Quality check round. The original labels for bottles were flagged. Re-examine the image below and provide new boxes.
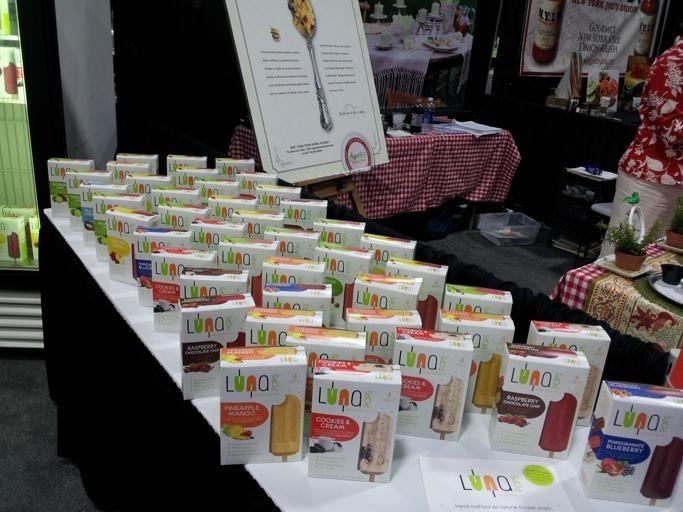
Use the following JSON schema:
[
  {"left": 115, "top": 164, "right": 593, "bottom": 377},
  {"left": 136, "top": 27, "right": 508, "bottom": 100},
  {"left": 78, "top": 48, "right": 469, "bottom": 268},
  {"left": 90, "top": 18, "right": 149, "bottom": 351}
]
[
  {"left": 413, "top": 98, "right": 433, "bottom": 133},
  {"left": 634, "top": 0, "right": 661, "bottom": 56},
  {"left": 531, "top": 1, "right": 566, "bottom": 64}
]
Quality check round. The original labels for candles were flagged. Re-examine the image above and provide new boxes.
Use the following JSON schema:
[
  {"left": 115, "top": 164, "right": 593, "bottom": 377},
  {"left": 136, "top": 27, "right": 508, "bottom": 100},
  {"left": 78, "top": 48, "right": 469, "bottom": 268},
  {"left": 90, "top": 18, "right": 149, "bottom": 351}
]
[
  {"left": 375, "top": 4, "right": 384, "bottom": 16},
  {"left": 431, "top": 2, "right": 440, "bottom": 16},
  {"left": 396, "top": 0, "right": 404, "bottom": 5}
]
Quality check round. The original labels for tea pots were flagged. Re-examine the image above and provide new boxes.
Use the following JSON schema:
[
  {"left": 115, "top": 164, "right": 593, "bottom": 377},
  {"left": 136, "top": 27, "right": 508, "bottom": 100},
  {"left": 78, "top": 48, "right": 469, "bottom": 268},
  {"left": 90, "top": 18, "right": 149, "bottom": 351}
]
[{"left": 456, "top": 5, "right": 475, "bottom": 38}]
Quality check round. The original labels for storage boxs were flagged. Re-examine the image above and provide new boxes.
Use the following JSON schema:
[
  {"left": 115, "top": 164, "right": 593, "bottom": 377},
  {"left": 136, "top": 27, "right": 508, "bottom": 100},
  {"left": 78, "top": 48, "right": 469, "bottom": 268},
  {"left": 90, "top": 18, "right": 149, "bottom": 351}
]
[{"left": 478, "top": 213, "right": 542, "bottom": 248}]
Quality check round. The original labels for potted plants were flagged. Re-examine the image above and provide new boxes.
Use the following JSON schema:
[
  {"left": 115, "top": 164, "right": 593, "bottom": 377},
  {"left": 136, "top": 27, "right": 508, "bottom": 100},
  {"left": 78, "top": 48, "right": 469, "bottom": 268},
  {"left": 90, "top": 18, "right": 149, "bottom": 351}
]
[
  {"left": 661, "top": 194, "right": 683, "bottom": 249},
  {"left": 598, "top": 217, "right": 663, "bottom": 271}
]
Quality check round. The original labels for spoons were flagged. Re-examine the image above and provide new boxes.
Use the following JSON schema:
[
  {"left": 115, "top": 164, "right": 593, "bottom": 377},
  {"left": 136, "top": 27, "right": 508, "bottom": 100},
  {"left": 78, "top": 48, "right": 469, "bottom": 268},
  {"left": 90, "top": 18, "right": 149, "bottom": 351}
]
[{"left": 288, "top": 0, "right": 333, "bottom": 131}]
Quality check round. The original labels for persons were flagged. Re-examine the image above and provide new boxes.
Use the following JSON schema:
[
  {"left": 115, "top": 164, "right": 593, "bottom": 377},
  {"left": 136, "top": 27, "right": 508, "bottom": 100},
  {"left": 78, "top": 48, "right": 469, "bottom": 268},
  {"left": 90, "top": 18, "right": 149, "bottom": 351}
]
[{"left": 596, "top": 31, "right": 683, "bottom": 260}]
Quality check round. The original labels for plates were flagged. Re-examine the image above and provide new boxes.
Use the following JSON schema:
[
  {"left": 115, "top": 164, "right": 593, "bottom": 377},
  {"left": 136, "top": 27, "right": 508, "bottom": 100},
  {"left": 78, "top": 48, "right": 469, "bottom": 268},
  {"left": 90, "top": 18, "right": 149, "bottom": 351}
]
[
  {"left": 362, "top": 22, "right": 385, "bottom": 35},
  {"left": 422, "top": 35, "right": 458, "bottom": 52}
]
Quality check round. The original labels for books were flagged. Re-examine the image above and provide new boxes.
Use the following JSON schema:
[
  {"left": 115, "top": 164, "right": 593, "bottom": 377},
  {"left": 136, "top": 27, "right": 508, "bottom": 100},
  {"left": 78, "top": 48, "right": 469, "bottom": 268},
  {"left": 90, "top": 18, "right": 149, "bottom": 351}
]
[{"left": 450, "top": 118, "right": 502, "bottom": 138}]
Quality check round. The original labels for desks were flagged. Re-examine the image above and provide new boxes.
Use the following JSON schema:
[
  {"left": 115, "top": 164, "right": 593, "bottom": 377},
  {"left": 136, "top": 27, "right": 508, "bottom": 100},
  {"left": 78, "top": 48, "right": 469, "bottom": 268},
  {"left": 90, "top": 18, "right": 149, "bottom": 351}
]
[
  {"left": 236, "top": 121, "right": 509, "bottom": 231},
  {"left": 555, "top": 236, "right": 683, "bottom": 356},
  {"left": 42, "top": 201, "right": 679, "bottom": 511}
]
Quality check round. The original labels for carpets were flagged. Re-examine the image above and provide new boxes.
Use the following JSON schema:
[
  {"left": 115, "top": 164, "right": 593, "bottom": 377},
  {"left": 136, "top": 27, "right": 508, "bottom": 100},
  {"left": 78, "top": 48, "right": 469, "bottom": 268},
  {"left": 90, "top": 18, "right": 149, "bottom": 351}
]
[{"left": 418, "top": 229, "right": 577, "bottom": 295}]
